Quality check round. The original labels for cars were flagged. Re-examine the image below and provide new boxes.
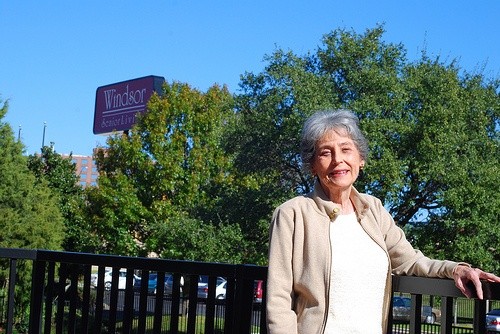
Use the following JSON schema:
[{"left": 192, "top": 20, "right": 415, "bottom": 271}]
[
  {"left": 90, "top": 268, "right": 142, "bottom": 295},
  {"left": 390, "top": 296, "right": 442, "bottom": 326},
  {"left": 486, "top": 307, "right": 500, "bottom": 334},
  {"left": 164, "top": 275, "right": 184, "bottom": 298},
  {"left": 133, "top": 273, "right": 168, "bottom": 298},
  {"left": 197, "top": 276, "right": 227, "bottom": 301}
]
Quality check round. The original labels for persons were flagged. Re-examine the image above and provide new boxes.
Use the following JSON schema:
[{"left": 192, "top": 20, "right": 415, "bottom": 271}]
[{"left": 264, "top": 111, "right": 500, "bottom": 334}]
